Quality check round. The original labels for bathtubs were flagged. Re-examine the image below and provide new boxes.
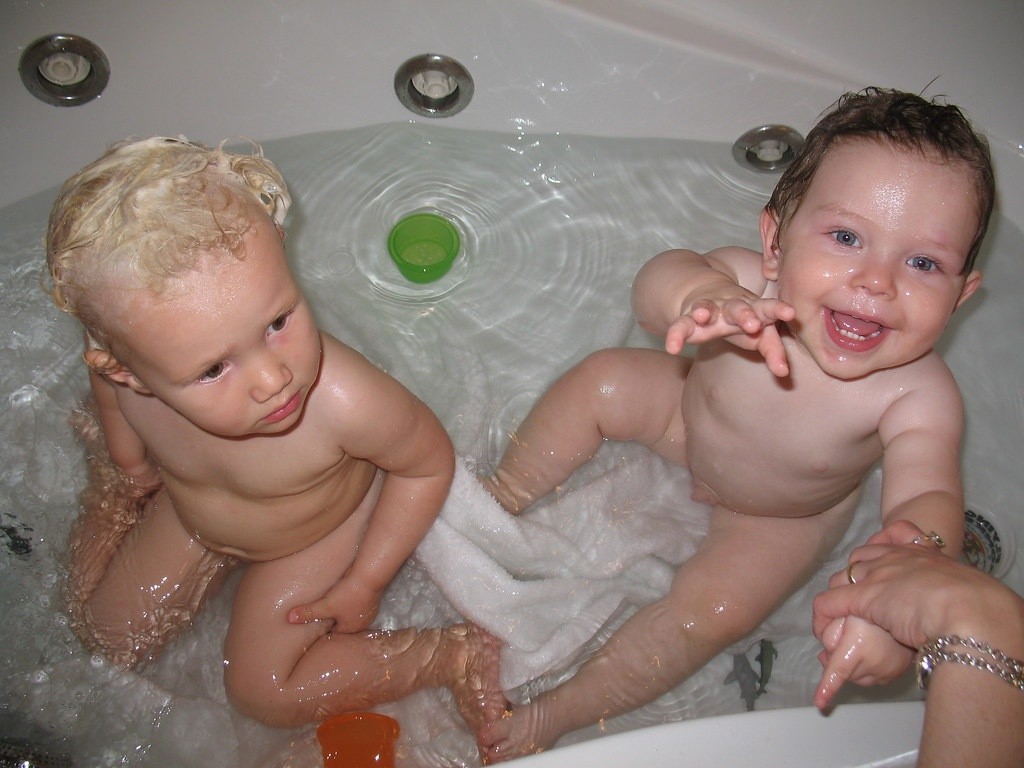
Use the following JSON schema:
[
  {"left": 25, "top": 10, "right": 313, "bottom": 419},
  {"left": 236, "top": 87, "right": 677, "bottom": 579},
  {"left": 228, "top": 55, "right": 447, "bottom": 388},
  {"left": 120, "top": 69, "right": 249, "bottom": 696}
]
[{"left": 2, "top": 0, "right": 1024, "bottom": 768}]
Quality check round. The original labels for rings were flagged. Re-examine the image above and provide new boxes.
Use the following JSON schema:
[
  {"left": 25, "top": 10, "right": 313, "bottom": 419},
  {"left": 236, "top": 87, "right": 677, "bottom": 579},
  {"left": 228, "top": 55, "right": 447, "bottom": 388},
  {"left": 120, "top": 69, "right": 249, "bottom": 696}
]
[
  {"left": 848, "top": 560, "right": 859, "bottom": 583},
  {"left": 911, "top": 532, "right": 944, "bottom": 547}
]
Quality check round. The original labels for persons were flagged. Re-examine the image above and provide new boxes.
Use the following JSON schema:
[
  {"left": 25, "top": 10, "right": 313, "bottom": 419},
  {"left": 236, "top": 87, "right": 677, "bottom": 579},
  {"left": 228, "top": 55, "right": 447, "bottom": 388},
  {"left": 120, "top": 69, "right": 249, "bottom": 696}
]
[
  {"left": 814, "top": 519, "right": 1024, "bottom": 768},
  {"left": 46, "top": 137, "right": 508, "bottom": 734},
  {"left": 472, "top": 84, "right": 996, "bottom": 767}
]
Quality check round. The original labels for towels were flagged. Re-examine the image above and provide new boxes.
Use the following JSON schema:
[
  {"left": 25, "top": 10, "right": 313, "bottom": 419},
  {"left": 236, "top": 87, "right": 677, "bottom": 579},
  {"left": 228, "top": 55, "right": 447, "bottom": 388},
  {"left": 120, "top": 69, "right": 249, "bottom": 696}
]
[{"left": 414, "top": 453, "right": 883, "bottom": 694}]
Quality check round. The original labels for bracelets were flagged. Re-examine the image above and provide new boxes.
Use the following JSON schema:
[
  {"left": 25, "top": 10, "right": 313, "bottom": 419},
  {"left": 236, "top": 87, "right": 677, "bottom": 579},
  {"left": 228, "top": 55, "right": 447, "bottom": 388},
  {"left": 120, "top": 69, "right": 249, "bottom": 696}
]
[{"left": 917, "top": 635, "right": 1023, "bottom": 697}]
